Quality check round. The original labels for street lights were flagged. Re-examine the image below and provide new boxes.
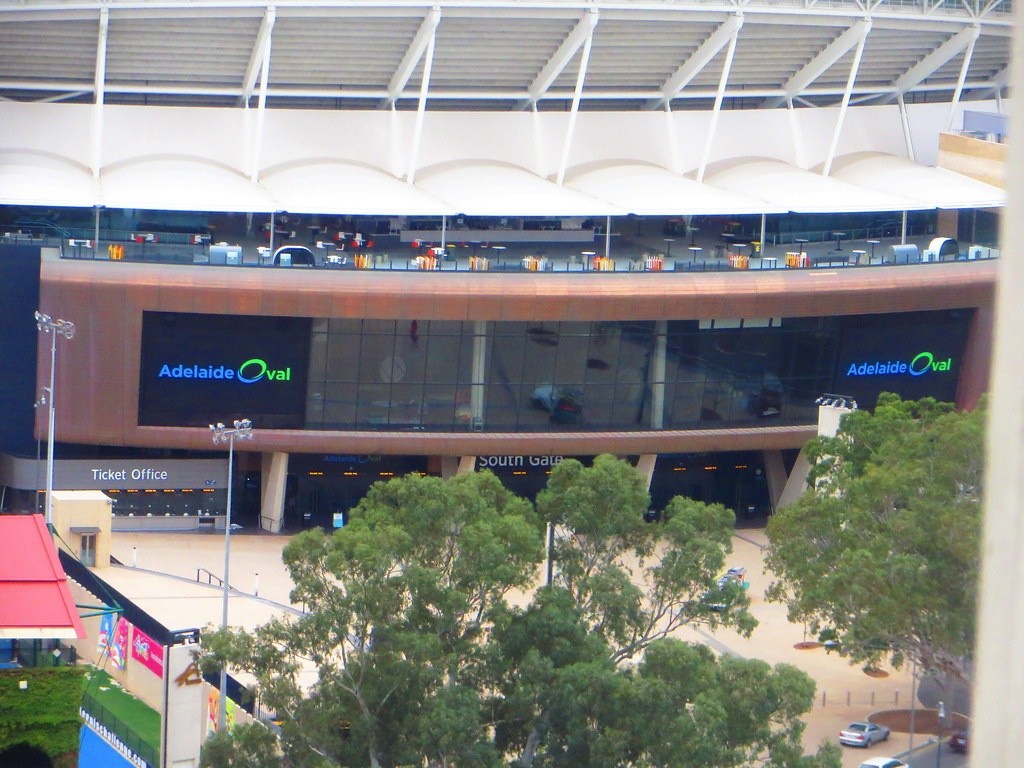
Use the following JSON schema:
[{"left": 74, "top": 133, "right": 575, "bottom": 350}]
[
  {"left": 821, "top": 640, "right": 917, "bottom": 768},
  {"left": 211, "top": 418, "right": 254, "bottom": 734},
  {"left": 33, "top": 310, "right": 76, "bottom": 524}
]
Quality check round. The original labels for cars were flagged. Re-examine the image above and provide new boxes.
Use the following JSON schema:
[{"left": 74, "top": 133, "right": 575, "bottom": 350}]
[
  {"left": 528, "top": 384, "right": 585, "bottom": 428},
  {"left": 948, "top": 730, "right": 968, "bottom": 754},
  {"left": 858, "top": 756, "right": 909, "bottom": 768},
  {"left": 702, "top": 566, "right": 746, "bottom": 612},
  {"left": 838, "top": 721, "right": 890, "bottom": 750}
]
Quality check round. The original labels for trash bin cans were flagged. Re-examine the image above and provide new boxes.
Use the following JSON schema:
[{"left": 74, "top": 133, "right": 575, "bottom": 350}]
[
  {"left": 208, "top": 243, "right": 243, "bottom": 265},
  {"left": 750, "top": 241, "right": 761, "bottom": 258},
  {"left": 280, "top": 253, "right": 291, "bottom": 267},
  {"left": 447, "top": 244, "right": 455, "bottom": 259}
]
